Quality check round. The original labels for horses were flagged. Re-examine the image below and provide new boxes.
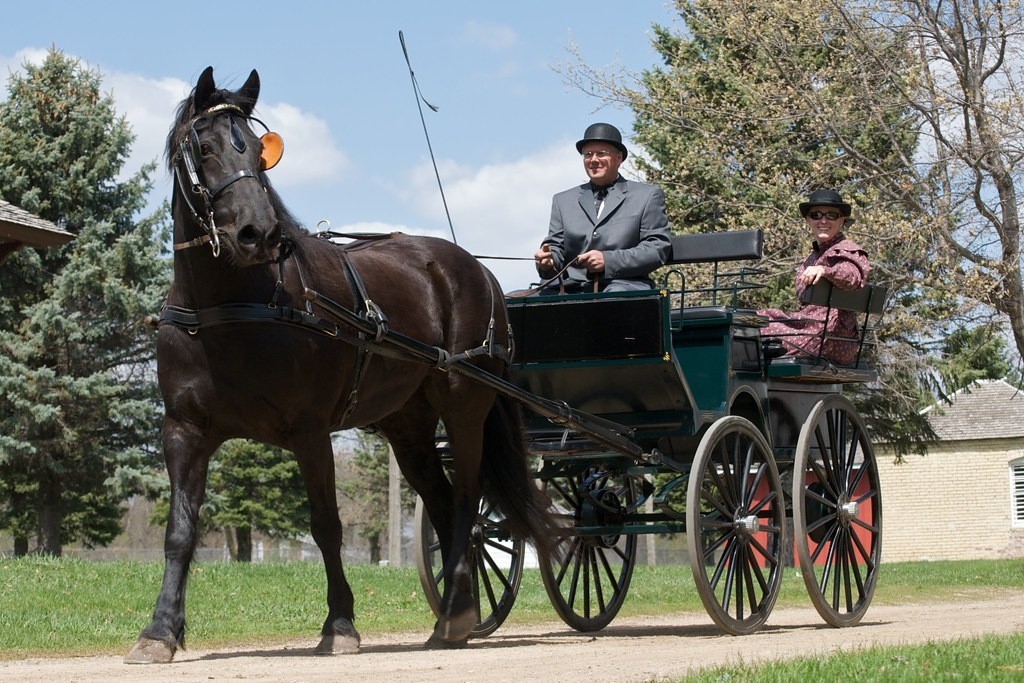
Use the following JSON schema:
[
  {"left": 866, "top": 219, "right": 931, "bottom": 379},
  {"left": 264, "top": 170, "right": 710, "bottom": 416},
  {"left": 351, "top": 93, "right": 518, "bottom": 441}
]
[{"left": 124, "top": 64, "right": 514, "bottom": 668}]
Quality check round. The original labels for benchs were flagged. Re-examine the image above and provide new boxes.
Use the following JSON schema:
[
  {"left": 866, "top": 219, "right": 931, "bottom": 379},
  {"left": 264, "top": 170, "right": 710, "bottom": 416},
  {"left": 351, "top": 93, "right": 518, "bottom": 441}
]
[
  {"left": 669, "top": 230, "right": 773, "bottom": 371},
  {"left": 736, "top": 276, "right": 890, "bottom": 383}
]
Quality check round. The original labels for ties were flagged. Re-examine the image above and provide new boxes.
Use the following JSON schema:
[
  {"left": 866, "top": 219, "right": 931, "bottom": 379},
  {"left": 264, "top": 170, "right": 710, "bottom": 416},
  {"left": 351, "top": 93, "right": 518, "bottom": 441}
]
[{"left": 592, "top": 189, "right": 604, "bottom": 215}]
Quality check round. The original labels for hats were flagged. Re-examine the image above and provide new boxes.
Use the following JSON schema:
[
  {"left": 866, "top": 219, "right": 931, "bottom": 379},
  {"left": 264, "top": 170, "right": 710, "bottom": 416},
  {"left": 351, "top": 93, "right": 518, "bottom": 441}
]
[
  {"left": 798, "top": 189, "right": 851, "bottom": 217},
  {"left": 576, "top": 123, "right": 628, "bottom": 162}
]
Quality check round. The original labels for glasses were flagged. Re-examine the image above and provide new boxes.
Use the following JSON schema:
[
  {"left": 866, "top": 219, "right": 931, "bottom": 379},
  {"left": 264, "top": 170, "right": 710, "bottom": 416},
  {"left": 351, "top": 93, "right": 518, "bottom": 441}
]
[
  {"left": 807, "top": 211, "right": 842, "bottom": 220},
  {"left": 581, "top": 150, "right": 617, "bottom": 160}
]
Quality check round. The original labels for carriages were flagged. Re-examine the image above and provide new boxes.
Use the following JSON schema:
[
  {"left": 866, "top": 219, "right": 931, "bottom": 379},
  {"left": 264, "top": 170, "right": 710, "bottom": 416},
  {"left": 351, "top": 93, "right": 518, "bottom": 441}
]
[{"left": 130, "top": 69, "right": 884, "bottom": 666}]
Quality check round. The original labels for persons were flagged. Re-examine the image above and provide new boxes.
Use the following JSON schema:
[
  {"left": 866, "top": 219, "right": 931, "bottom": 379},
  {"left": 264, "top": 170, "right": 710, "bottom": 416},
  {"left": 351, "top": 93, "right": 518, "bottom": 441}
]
[
  {"left": 505, "top": 123, "right": 672, "bottom": 300},
  {"left": 757, "top": 191, "right": 870, "bottom": 364}
]
